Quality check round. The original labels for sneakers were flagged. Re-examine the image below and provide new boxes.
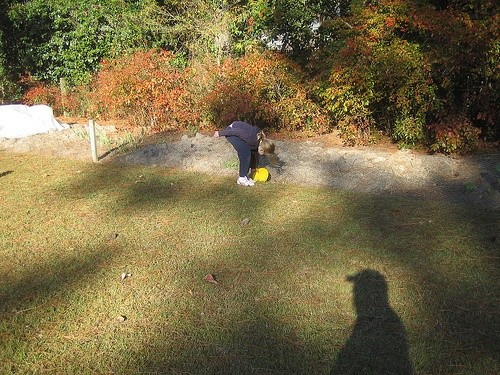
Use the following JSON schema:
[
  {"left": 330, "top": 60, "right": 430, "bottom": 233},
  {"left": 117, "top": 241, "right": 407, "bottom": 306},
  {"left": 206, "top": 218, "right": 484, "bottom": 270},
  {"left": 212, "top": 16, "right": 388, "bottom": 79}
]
[{"left": 236, "top": 176, "right": 256, "bottom": 186}]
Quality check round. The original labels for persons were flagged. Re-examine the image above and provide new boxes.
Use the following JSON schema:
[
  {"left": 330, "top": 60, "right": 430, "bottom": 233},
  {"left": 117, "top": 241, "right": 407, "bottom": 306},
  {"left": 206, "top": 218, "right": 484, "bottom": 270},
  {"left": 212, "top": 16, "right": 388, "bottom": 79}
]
[{"left": 212, "top": 121, "right": 279, "bottom": 187}]
